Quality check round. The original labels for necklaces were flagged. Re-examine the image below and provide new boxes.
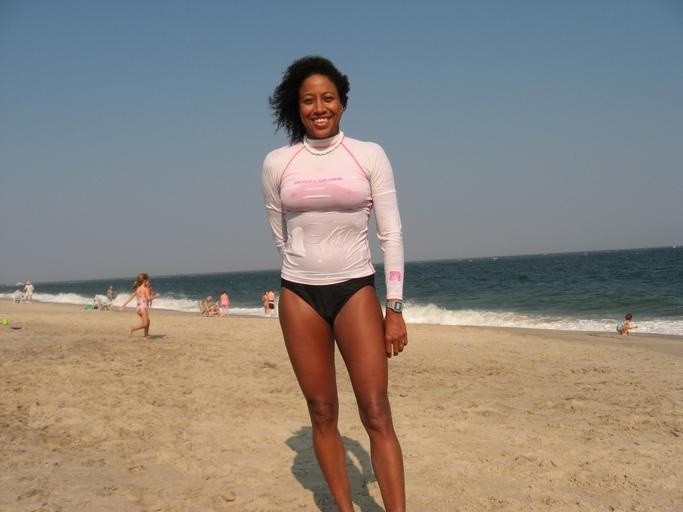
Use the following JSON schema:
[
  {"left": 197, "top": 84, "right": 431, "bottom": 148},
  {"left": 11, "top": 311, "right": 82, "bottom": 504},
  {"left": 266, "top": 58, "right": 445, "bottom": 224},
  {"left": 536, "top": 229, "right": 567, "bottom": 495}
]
[{"left": 302, "top": 134, "right": 343, "bottom": 155}]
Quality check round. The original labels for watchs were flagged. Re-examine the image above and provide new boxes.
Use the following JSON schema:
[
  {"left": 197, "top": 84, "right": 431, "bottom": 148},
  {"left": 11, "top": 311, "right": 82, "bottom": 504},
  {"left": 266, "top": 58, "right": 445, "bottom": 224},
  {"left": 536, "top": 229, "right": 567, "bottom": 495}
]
[{"left": 385, "top": 299, "right": 406, "bottom": 313}]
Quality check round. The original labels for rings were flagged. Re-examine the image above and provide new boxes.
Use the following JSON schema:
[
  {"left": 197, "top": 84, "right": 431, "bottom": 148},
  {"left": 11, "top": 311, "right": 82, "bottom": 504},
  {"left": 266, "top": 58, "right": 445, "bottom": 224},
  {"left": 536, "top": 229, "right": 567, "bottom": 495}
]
[{"left": 398, "top": 344, "right": 404, "bottom": 347}]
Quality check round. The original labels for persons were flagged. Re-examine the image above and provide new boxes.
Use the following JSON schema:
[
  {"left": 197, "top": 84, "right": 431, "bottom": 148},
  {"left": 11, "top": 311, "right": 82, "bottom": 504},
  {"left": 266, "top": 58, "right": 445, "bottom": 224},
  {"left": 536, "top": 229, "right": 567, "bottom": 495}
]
[
  {"left": 616, "top": 314, "right": 637, "bottom": 336},
  {"left": 146, "top": 279, "right": 153, "bottom": 308},
  {"left": 118, "top": 273, "right": 159, "bottom": 339},
  {"left": 218, "top": 291, "right": 229, "bottom": 317},
  {"left": 23, "top": 281, "right": 33, "bottom": 303},
  {"left": 104, "top": 286, "right": 115, "bottom": 309},
  {"left": 264, "top": 55, "right": 412, "bottom": 512},
  {"left": 200, "top": 296, "right": 213, "bottom": 316},
  {"left": 201, "top": 302, "right": 221, "bottom": 314},
  {"left": 267, "top": 287, "right": 273, "bottom": 315},
  {"left": 261, "top": 291, "right": 267, "bottom": 313}
]
